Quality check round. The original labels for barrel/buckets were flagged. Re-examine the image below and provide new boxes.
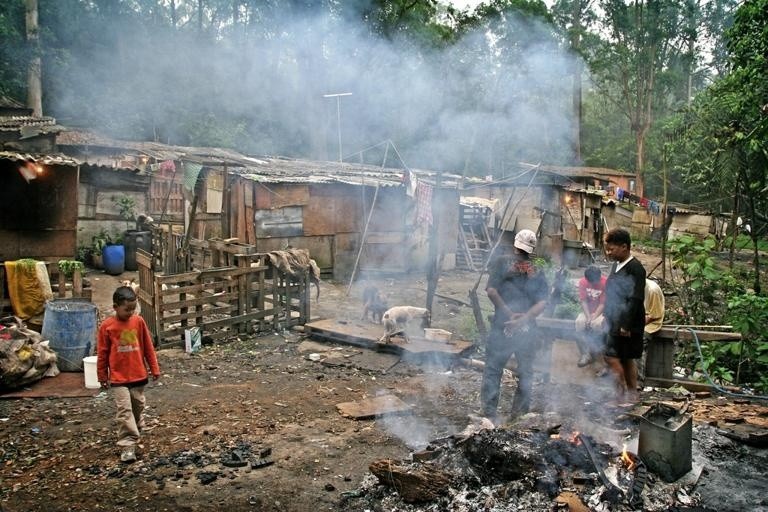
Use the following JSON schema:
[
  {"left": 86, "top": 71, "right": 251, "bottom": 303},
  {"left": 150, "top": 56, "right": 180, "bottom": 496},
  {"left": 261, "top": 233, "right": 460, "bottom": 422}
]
[
  {"left": 125, "top": 229, "right": 152, "bottom": 270},
  {"left": 82, "top": 356, "right": 110, "bottom": 389},
  {"left": 103, "top": 244, "right": 125, "bottom": 275},
  {"left": 40, "top": 298, "right": 98, "bottom": 371},
  {"left": 92, "top": 254, "right": 103, "bottom": 269},
  {"left": 563, "top": 239, "right": 583, "bottom": 269}
]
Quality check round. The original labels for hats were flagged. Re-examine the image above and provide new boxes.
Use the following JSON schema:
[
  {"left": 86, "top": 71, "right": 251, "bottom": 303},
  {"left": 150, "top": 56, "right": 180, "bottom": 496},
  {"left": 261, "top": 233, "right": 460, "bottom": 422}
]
[{"left": 513, "top": 229, "right": 536, "bottom": 254}]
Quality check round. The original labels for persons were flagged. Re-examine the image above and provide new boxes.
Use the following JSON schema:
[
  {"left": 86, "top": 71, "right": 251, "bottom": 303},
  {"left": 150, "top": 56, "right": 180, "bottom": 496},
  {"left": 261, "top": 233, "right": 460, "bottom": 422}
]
[
  {"left": 574, "top": 267, "right": 608, "bottom": 376},
  {"left": 604, "top": 228, "right": 666, "bottom": 402},
  {"left": 480, "top": 229, "right": 548, "bottom": 425},
  {"left": 97, "top": 286, "right": 160, "bottom": 464}
]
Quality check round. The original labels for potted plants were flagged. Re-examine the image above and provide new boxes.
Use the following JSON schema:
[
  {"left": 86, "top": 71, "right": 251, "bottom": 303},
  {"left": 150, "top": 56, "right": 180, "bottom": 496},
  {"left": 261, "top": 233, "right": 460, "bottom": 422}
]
[{"left": 91, "top": 227, "right": 113, "bottom": 270}]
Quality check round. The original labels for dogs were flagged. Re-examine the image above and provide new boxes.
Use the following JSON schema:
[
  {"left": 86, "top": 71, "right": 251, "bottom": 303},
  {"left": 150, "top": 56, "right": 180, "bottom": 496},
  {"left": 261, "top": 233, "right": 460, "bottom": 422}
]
[
  {"left": 357, "top": 279, "right": 392, "bottom": 324},
  {"left": 381, "top": 305, "right": 431, "bottom": 345}
]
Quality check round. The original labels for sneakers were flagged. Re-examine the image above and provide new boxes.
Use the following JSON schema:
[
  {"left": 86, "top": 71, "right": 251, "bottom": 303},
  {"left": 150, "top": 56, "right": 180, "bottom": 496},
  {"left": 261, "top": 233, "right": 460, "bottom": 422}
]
[
  {"left": 468, "top": 408, "right": 495, "bottom": 419},
  {"left": 120, "top": 446, "right": 136, "bottom": 462},
  {"left": 603, "top": 395, "right": 641, "bottom": 410},
  {"left": 577, "top": 353, "right": 593, "bottom": 367}
]
